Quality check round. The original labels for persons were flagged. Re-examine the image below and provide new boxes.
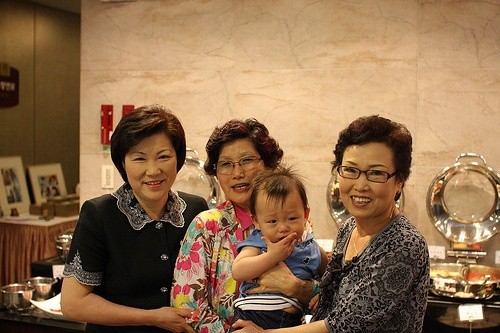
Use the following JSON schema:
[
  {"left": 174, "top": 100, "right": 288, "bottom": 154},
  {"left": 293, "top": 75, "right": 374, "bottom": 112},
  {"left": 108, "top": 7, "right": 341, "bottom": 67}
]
[
  {"left": 231, "top": 162, "right": 322, "bottom": 331},
  {"left": 170, "top": 117, "right": 329, "bottom": 333},
  {"left": 60, "top": 105, "right": 212, "bottom": 333},
  {"left": 230, "top": 115, "right": 430, "bottom": 332}
]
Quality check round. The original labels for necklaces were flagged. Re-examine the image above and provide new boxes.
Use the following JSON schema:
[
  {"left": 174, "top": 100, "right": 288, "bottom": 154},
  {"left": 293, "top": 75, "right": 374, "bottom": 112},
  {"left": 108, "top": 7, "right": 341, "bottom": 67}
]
[{"left": 352, "top": 206, "right": 395, "bottom": 257}]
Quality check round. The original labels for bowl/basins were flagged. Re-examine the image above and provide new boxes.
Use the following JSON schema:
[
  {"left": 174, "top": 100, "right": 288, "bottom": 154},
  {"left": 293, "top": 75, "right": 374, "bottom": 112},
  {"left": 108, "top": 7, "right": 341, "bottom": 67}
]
[
  {"left": 0, "top": 283, "right": 36, "bottom": 314},
  {"left": 22, "top": 276, "right": 58, "bottom": 301},
  {"left": 54, "top": 228, "right": 75, "bottom": 262}
]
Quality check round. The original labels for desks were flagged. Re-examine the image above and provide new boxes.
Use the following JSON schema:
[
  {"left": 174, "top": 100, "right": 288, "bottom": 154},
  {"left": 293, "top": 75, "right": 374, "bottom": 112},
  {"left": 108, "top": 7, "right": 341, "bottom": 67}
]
[
  {"left": 0, "top": 214, "right": 80, "bottom": 287},
  {"left": 0, "top": 301, "right": 88, "bottom": 331}
]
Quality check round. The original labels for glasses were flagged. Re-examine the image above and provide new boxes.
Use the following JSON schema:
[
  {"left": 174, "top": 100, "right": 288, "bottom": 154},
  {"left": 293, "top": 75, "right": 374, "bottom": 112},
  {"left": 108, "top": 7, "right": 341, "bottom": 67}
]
[
  {"left": 212, "top": 155, "right": 263, "bottom": 174},
  {"left": 336, "top": 165, "right": 397, "bottom": 184}
]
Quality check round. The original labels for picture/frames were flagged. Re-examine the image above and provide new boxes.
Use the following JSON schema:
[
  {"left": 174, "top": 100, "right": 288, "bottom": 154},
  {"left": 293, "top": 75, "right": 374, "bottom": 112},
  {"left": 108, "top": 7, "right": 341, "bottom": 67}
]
[
  {"left": 0, "top": 156, "right": 32, "bottom": 220},
  {"left": 28, "top": 163, "right": 67, "bottom": 207}
]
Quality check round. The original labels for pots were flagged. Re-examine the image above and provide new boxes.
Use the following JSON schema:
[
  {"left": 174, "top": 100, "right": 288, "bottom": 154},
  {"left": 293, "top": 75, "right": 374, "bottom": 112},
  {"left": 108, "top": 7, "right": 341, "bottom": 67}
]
[{"left": 427, "top": 263, "right": 500, "bottom": 301}]
